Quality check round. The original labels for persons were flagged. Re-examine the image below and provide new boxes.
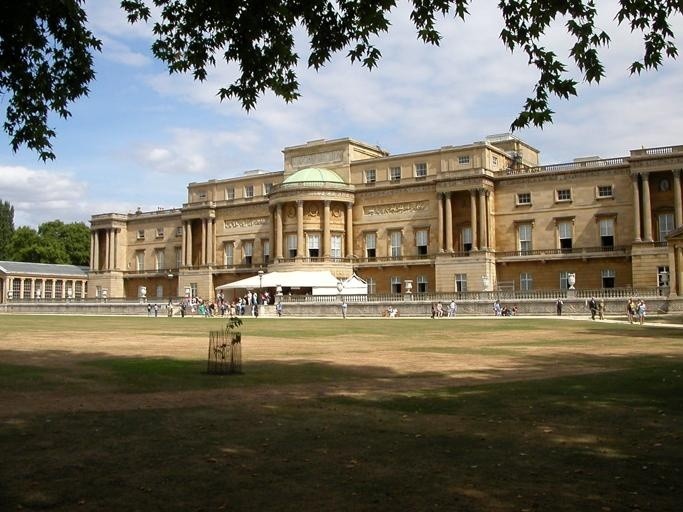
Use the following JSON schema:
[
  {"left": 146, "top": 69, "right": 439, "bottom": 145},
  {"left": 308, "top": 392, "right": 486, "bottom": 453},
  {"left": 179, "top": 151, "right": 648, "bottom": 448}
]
[
  {"left": 338, "top": 301, "right": 347, "bottom": 320},
  {"left": 429, "top": 294, "right": 647, "bottom": 327},
  {"left": 143, "top": 287, "right": 283, "bottom": 319},
  {"left": 389, "top": 307, "right": 397, "bottom": 317},
  {"left": 387, "top": 303, "right": 392, "bottom": 317}
]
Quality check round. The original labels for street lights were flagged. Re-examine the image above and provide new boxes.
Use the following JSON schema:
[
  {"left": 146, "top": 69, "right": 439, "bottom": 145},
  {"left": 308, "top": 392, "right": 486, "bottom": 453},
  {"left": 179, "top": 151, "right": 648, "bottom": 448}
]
[
  {"left": 168, "top": 268, "right": 173, "bottom": 303},
  {"left": 258, "top": 265, "right": 264, "bottom": 305}
]
